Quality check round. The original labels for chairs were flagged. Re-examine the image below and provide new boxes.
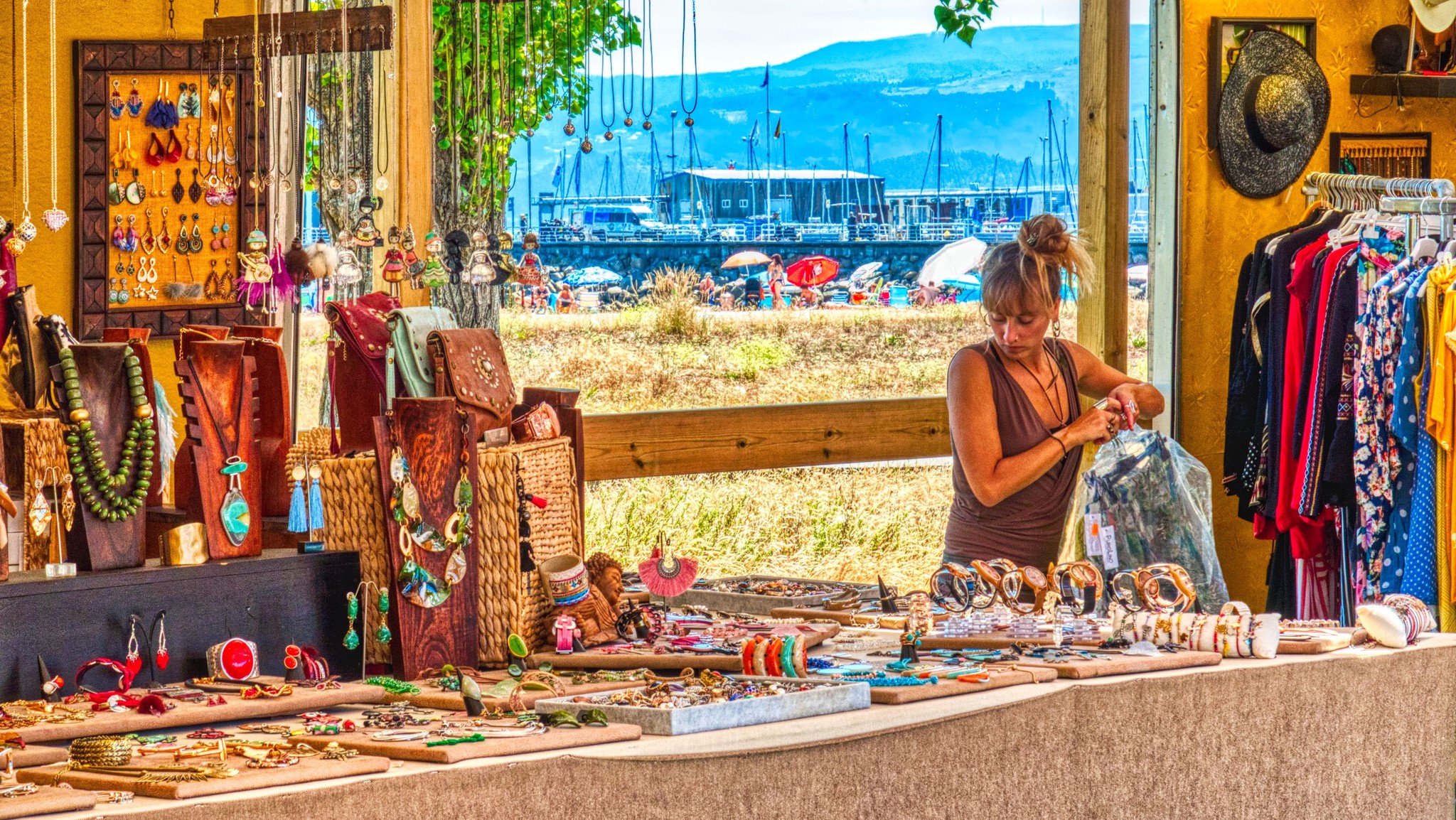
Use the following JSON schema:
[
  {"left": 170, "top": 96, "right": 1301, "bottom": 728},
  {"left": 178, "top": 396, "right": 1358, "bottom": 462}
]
[{"left": 577, "top": 279, "right": 908, "bottom": 308}]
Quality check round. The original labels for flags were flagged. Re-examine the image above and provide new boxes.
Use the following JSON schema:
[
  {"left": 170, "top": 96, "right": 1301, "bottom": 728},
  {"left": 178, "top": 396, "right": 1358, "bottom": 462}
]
[
  {"left": 773, "top": 118, "right": 780, "bottom": 139},
  {"left": 761, "top": 61, "right": 768, "bottom": 87}
]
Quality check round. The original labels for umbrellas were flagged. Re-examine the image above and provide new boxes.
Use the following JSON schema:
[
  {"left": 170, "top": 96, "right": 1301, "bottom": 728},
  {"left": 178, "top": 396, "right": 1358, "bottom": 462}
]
[
  {"left": 563, "top": 266, "right": 622, "bottom": 285},
  {"left": 722, "top": 236, "right": 987, "bottom": 305}
]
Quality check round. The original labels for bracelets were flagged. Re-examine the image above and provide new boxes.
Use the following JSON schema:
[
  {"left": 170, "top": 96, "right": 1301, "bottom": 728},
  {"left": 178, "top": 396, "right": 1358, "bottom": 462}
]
[
  {"left": 69, "top": 659, "right": 131, "bottom": 764},
  {"left": 510, "top": 554, "right": 664, "bottom": 713},
  {"left": 1120, "top": 594, "right": 1430, "bottom": 659},
  {"left": 1050, "top": 436, "right": 1067, "bottom": 461}
]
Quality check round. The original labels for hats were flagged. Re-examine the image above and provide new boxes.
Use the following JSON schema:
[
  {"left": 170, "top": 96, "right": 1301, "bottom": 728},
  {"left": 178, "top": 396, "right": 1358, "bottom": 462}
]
[
  {"left": 1370, "top": 23, "right": 1421, "bottom": 73},
  {"left": 1217, "top": 26, "right": 1331, "bottom": 199},
  {"left": 704, "top": 272, "right": 712, "bottom": 278},
  {"left": 1409, "top": 0, "right": 1456, "bottom": 33}
]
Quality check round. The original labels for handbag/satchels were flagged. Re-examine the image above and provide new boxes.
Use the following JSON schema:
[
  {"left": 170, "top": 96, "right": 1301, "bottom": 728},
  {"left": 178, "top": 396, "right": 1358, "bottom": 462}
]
[
  {"left": 427, "top": 327, "right": 518, "bottom": 442},
  {"left": 7, "top": 283, "right": 53, "bottom": 409},
  {"left": 322, "top": 291, "right": 407, "bottom": 456},
  {"left": 384, "top": 306, "right": 459, "bottom": 409},
  {"left": 35, "top": 313, "right": 80, "bottom": 364},
  {"left": 0, "top": 220, "right": 18, "bottom": 353}
]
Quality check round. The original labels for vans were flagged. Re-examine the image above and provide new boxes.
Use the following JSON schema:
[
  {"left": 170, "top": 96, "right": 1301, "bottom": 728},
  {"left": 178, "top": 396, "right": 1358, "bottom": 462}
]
[{"left": 584, "top": 204, "right": 668, "bottom": 242}]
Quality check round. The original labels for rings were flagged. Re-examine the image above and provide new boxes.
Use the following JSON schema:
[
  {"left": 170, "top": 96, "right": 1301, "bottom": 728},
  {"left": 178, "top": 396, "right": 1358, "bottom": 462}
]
[
  {"left": 206, "top": 637, "right": 303, "bottom": 683},
  {"left": 460, "top": 633, "right": 529, "bottom": 701},
  {"left": 1092, "top": 396, "right": 1136, "bottom": 434},
  {"left": 158, "top": 522, "right": 210, "bottom": 565},
  {"left": 39, "top": 675, "right": 64, "bottom": 696},
  {"left": 511, "top": 400, "right": 561, "bottom": 443},
  {"left": 878, "top": 587, "right": 922, "bottom": 646}
]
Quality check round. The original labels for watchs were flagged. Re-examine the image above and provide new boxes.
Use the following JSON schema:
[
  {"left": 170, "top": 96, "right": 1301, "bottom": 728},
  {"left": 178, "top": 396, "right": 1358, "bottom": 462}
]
[{"left": 931, "top": 557, "right": 1196, "bottom": 618}]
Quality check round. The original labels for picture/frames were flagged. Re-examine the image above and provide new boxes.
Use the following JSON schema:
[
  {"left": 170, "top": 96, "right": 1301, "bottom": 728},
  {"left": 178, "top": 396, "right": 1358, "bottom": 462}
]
[{"left": 1208, "top": 16, "right": 1316, "bottom": 149}]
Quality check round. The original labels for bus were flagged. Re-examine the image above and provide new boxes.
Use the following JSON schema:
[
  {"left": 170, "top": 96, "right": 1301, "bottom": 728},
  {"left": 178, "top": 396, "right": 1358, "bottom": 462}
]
[{"left": 530, "top": 195, "right": 672, "bottom": 240}]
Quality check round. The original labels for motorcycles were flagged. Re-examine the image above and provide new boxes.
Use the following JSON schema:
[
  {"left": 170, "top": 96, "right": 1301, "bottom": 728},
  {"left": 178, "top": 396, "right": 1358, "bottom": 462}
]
[{"left": 562, "top": 223, "right": 585, "bottom": 241}]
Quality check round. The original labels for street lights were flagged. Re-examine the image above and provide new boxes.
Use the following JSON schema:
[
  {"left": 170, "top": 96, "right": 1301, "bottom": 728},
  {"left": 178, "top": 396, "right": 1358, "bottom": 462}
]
[
  {"left": 1039, "top": 136, "right": 1048, "bottom": 215},
  {"left": 669, "top": 110, "right": 678, "bottom": 224}
]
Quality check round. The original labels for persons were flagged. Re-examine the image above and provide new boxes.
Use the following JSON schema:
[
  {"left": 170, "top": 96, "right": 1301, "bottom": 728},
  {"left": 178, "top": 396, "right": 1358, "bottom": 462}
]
[
  {"left": 545, "top": 551, "right": 623, "bottom": 648},
  {"left": 520, "top": 217, "right": 564, "bottom": 237},
  {"left": 771, "top": 212, "right": 783, "bottom": 242},
  {"left": 847, "top": 212, "right": 858, "bottom": 242},
  {"left": 239, "top": 196, "right": 544, "bottom": 314},
  {"left": 937, "top": 215, "right": 1165, "bottom": 601},
  {"left": 535, "top": 279, "right": 575, "bottom": 314},
  {"left": 700, "top": 255, "right": 963, "bottom": 310}
]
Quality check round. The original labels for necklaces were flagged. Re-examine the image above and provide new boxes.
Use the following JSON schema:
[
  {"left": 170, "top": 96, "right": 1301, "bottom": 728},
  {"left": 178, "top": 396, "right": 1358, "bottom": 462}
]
[
  {"left": 383, "top": 404, "right": 475, "bottom": 608},
  {"left": 0, "top": 700, "right": 239, "bottom": 783},
  {"left": 33, "top": 316, "right": 280, "bottom": 547},
  {"left": 5, "top": 0, "right": 697, "bottom": 295},
  {"left": 1016, "top": 344, "right": 1067, "bottom": 429}
]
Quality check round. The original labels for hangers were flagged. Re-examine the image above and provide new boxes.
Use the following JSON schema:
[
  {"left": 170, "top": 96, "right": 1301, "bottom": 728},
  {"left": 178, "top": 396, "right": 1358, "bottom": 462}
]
[{"left": 1316, "top": 172, "right": 1456, "bottom": 260}]
